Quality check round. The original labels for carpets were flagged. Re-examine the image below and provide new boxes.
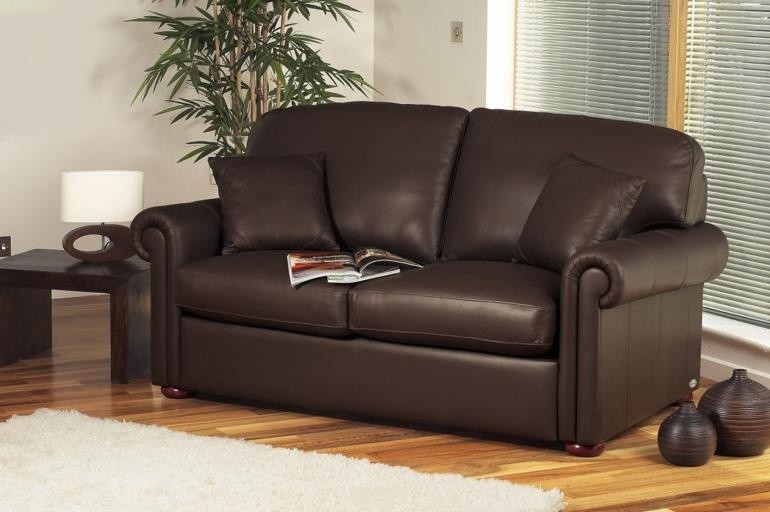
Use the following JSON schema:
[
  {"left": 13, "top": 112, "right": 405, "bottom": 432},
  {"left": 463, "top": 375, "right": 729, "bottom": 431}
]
[{"left": 0, "top": 406, "right": 566, "bottom": 510}]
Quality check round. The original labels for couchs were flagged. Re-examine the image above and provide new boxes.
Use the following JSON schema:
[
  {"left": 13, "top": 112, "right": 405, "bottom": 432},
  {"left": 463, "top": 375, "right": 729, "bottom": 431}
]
[{"left": 130, "top": 101, "right": 729, "bottom": 457}]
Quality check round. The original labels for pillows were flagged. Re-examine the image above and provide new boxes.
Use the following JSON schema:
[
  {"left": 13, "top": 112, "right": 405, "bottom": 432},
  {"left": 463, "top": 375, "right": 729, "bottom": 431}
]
[
  {"left": 517, "top": 155, "right": 645, "bottom": 268},
  {"left": 207, "top": 156, "right": 338, "bottom": 254}
]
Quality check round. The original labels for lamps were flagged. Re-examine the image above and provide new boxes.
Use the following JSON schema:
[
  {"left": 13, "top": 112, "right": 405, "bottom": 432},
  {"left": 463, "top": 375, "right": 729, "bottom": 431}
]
[{"left": 58, "top": 170, "right": 145, "bottom": 264}]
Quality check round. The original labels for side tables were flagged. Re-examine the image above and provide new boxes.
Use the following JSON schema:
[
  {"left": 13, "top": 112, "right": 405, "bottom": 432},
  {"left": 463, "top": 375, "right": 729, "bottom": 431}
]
[{"left": 0, "top": 248, "right": 151, "bottom": 384}]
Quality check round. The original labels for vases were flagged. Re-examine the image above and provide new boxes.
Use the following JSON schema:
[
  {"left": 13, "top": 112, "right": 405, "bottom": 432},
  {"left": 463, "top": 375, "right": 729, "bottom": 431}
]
[
  {"left": 697, "top": 369, "right": 770, "bottom": 457},
  {"left": 658, "top": 401, "right": 718, "bottom": 466}
]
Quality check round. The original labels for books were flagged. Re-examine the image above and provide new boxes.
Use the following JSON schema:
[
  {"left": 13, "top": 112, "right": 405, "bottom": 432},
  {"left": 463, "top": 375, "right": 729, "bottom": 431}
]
[
  {"left": 285, "top": 244, "right": 425, "bottom": 289},
  {"left": 326, "top": 260, "right": 402, "bottom": 285}
]
